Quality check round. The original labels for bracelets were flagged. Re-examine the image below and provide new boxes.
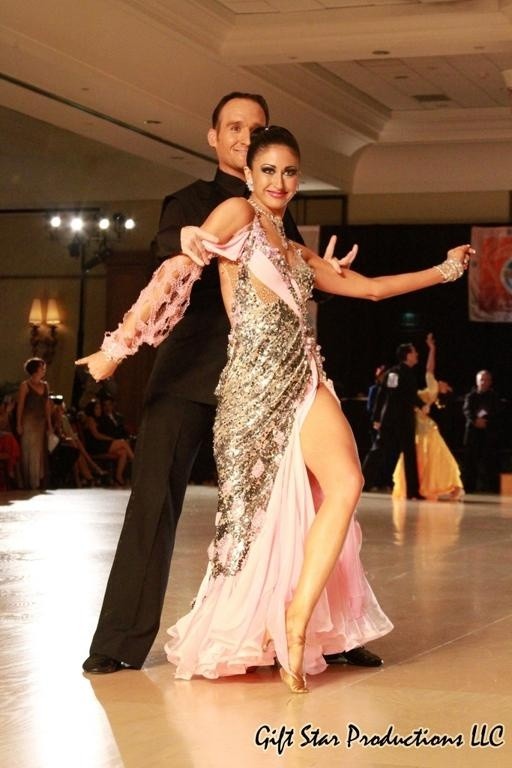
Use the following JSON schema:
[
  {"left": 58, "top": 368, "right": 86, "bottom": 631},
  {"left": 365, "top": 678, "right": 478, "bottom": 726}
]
[{"left": 434, "top": 259, "right": 464, "bottom": 283}]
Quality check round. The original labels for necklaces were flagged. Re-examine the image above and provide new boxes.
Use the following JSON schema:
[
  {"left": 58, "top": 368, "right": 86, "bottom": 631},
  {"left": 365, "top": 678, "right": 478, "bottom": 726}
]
[{"left": 247, "top": 196, "right": 288, "bottom": 249}]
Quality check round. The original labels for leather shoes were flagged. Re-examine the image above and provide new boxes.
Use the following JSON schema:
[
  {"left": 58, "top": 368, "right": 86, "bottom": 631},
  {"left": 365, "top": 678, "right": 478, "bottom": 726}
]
[
  {"left": 82, "top": 654, "right": 123, "bottom": 675},
  {"left": 322, "top": 646, "right": 384, "bottom": 667}
]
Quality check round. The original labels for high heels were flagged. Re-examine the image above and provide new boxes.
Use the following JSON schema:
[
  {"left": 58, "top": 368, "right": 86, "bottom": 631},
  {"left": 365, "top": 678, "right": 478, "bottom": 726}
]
[{"left": 262, "top": 609, "right": 310, "bottom": 694}]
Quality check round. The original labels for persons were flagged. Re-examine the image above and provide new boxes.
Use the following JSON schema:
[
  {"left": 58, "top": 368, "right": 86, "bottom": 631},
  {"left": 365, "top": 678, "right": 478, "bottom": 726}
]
[
  {"left": 80, "top": 89, "right": 383, "bottom": 674},
  {"left": 1, "top": 357, "right": 137, "bottom": 489},
  {"left": 338, "top": 333, "right": 501, "bottom": 504},
  {"left": 74, "top": 123, "right": 477, "bottom": 693}
]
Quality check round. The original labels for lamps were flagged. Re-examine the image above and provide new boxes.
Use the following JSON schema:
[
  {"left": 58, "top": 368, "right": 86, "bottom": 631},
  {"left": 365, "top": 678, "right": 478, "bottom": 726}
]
[
  {"left": 26, "top": 293, "right": 64, "bottom": 367},
  {"left": 415, "top": 88, "right": 447, "bottom": 112},
  {"left": 44, "top": 209, "right": 138, "bottom": 254}
]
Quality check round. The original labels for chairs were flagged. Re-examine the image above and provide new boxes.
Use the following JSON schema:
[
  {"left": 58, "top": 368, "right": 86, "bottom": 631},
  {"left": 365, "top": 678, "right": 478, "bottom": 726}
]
[{"left": 90, "top": 451, "right": 132, "bottom": 484}]
